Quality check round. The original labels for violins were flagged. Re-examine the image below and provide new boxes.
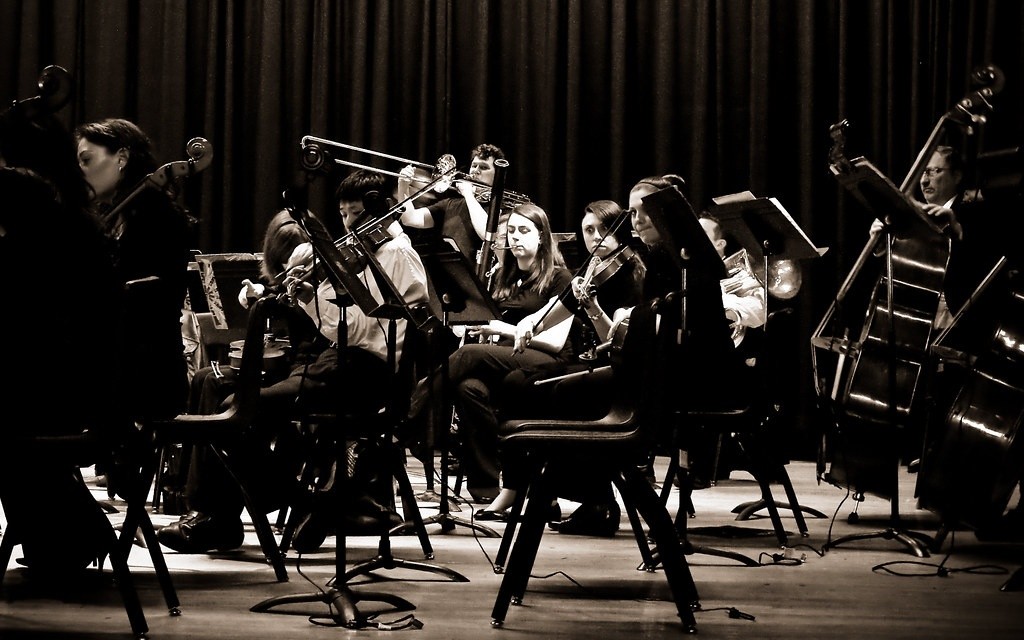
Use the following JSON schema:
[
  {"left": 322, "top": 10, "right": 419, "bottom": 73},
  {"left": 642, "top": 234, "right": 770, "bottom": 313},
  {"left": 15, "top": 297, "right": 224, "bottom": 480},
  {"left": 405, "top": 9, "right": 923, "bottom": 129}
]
[
  {"left": 277, "top": 226, "right": 394, "bottom": 310},
  {"left": 576, "top": 245, "right": 634, "bottom": 312},
  {"left": 576, "top": 307, "right": 631, "bottom": 363}
]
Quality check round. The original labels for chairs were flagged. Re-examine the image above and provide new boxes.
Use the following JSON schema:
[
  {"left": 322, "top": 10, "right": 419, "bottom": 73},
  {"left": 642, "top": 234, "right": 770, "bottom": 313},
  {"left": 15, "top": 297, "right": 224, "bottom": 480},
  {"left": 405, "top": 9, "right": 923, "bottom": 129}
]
[{"left": 0, "top": 288, "right": 808, "bottom": 634}]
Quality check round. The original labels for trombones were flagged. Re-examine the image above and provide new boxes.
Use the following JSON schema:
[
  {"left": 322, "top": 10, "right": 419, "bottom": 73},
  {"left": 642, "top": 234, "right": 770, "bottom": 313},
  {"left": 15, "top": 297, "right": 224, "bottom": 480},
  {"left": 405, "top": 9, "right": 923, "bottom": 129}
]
[{"left": 299, "top": 131, "right": 531, "bottom": 210}]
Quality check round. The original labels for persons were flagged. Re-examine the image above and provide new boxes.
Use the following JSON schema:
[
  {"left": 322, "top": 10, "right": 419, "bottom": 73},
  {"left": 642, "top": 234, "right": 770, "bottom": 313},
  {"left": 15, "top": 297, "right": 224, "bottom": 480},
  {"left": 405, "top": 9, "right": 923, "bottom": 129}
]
[
  {"left": 0, "top": 73, "right": 194, "bottom": 578},
  {"left": 156, "top": 143, "right": 767, "bottom": 551},
  {"left": 869, "top": 141, "right": 1024, "bottom": 544}
]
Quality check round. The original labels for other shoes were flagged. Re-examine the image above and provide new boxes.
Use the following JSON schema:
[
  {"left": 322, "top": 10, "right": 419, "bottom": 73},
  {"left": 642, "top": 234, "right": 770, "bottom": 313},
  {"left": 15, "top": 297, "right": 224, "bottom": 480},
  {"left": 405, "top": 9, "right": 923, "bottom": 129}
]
[
  {"left": 157, "top": 508, "right": 245, "bottom": 554},
  {"left": 474, "top": 502, "right": 516, "bottom": 519},
  {"left": 638, "top": 464, "right": 655, "bottom": 482},
  {"left": 548, "top": 499, "right": 620, "bottom": 536},
  {"left": 675, "top": 475, "right": 711, "bottom": 490},
  {"left": 502, "top": 502, "right": 561, "bottom": 523}
]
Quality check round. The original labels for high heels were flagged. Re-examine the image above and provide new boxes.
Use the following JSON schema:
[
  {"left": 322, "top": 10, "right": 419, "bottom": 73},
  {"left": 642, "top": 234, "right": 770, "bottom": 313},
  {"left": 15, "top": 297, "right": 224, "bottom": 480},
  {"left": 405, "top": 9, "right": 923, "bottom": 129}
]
[{"left": 19, "top": 514, "right": 116, "bottom": 576}]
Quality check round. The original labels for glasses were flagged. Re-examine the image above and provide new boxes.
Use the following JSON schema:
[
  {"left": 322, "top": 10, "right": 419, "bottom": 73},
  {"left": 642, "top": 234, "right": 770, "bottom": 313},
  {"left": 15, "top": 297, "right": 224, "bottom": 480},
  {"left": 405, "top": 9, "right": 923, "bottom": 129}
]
[{"left": 920, "top": 167, "right": 953, "bottom": 178}]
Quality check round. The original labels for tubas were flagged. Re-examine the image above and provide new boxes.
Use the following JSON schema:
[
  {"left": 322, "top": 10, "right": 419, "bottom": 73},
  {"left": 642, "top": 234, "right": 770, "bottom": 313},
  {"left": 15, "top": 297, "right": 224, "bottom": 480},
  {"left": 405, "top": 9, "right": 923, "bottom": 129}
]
[{"left": 721, "top": 232, "right": 804, "bottom": 349}]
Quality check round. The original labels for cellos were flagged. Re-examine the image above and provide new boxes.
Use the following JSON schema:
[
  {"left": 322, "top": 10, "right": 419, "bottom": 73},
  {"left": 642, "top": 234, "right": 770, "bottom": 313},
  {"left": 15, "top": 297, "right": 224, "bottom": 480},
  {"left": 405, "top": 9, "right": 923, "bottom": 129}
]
[
  {"left": 841, "top": 61, "right": 1005, "bottom": 431},
  {"left": 912, "top": 270, "right": 1024, "bottom": 539},
  {"left": 103, "top": 135, "right": 215, "bottom": 226}
]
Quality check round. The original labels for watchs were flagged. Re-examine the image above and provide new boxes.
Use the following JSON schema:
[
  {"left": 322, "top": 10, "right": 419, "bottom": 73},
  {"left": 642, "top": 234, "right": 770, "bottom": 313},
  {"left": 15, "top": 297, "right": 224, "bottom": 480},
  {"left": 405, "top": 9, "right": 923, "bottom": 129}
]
[{"left": 588, "top": 310, "right": 604, "bottom": 321}]
